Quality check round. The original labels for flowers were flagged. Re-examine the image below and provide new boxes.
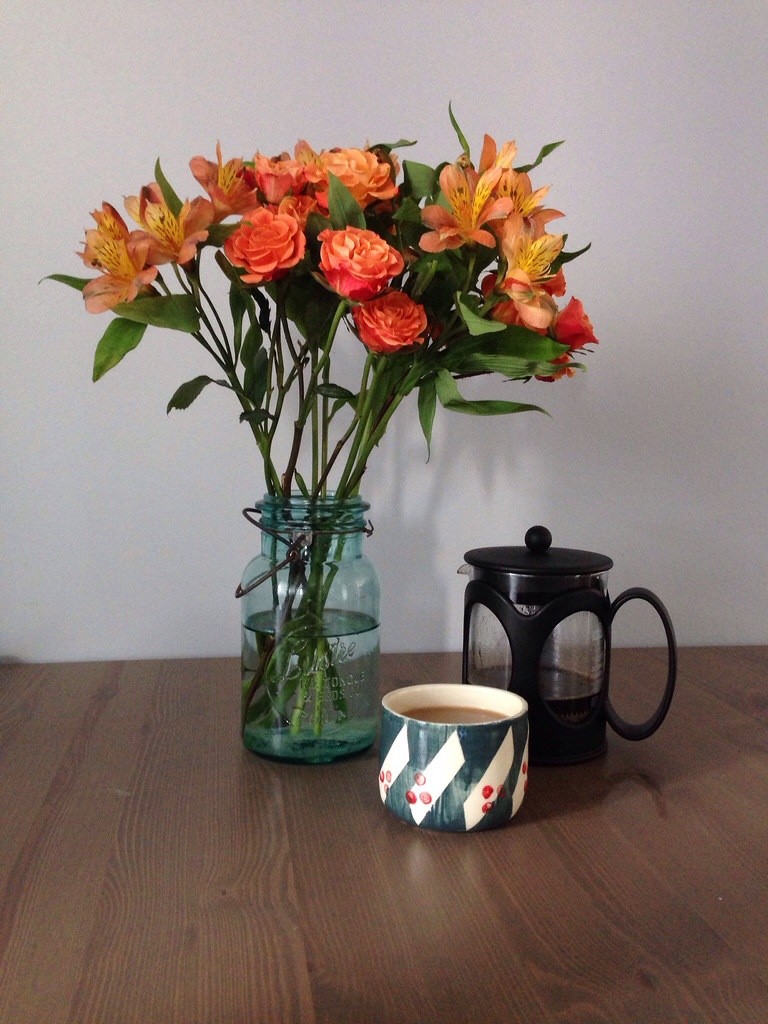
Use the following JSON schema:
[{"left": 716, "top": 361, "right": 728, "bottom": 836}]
[{"left": 35, "top": 100, "right": 600, "bottom": 743}]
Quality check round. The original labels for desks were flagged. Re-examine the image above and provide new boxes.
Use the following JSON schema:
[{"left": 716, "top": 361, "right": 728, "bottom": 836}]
[{"left": 0, "top": 643, "right": 768, "bottom": 1024}]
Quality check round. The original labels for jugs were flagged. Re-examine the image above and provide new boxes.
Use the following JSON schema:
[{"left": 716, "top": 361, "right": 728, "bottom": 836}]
[{"left": 455, "top": 526, "right": 679, "bottom": 768}]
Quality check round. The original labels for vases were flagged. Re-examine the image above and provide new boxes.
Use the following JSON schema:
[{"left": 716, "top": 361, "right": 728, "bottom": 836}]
[{"left": 235, "top": 490, "right": 381, "bottom": 762}]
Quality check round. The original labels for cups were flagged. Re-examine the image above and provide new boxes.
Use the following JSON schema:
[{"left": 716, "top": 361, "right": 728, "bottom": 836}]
[{"left": 379, "top": 682, "right": 531, "bottom": 833}]
